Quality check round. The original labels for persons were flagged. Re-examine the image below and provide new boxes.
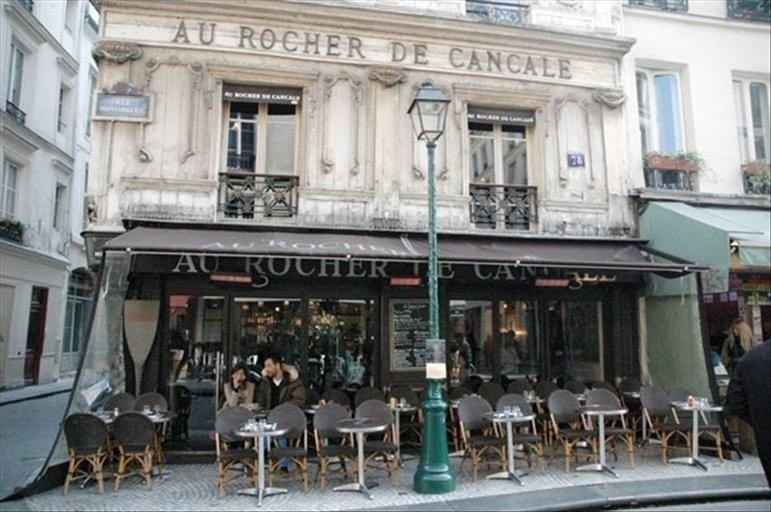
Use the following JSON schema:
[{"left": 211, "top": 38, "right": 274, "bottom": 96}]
[
  {"left": 724, "top": 338, "right": 770, "bottom": 488},
  {"left": 255, "top": 354, "right": 306, "bottom": 476},
  {"left": 178, "top": 342, "right": 209, "bottom": 380},
  {"left": 720, "top": 316, "right": 758, "bottom": 432},
  {"left": 450, "top": 328, "right": 524, "bottom": 376},
  {"left": 216, "top": 361, "right": 256, "bottom": 416}
]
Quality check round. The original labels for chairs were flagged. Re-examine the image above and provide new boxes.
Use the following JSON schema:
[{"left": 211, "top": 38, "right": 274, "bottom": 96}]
[
  {"left": 420, "top": 387, "right": 458, "bottom": 451},
  {"left": 59, "top": 393, "right": 179, "bottom": 496},
  {"left": 216, "top": 384, "right": 419, "bottom": 507},
  {"left": 449, "top": 374, "right": 724, "bottom": 486}
]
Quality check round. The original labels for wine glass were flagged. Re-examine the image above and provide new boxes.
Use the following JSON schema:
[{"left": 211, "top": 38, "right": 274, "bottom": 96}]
[{"left": 504, "top": 406, "right": 511, "bottom": 418}]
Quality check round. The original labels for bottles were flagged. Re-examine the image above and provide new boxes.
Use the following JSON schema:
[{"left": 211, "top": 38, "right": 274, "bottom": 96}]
[{"left": 688, "top": 393, "right": 693, "bottom": 406}]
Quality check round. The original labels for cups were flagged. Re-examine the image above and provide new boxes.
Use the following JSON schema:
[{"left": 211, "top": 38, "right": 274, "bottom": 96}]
[
  {"left": 96, "top": 404, "right": 163, "bottom": 419},
  {"left": 400, "top": 398, "right": 407, "bottom": 407},
  {"left": 390, "top": 397, "right": 397, "bottom": 408},
  {"left": 259, "top": 419, "right": 266, "bottom": 431},
  {"left": 248, "top": 418, "right": 254, "bottom": 431},
  {"left": 512, "top": 405, "right": 520, "bottom": 418},
  {"left": 529, "top": 391, "right": 535, "bottom": 399},
  {"left": 696, "top": 398, "right": 708, "bottom": 406}
]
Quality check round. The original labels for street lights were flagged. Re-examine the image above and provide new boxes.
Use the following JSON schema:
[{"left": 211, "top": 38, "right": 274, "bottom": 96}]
[{"left": 404, "top": 77, "right": 459, "bottom": 494}]
